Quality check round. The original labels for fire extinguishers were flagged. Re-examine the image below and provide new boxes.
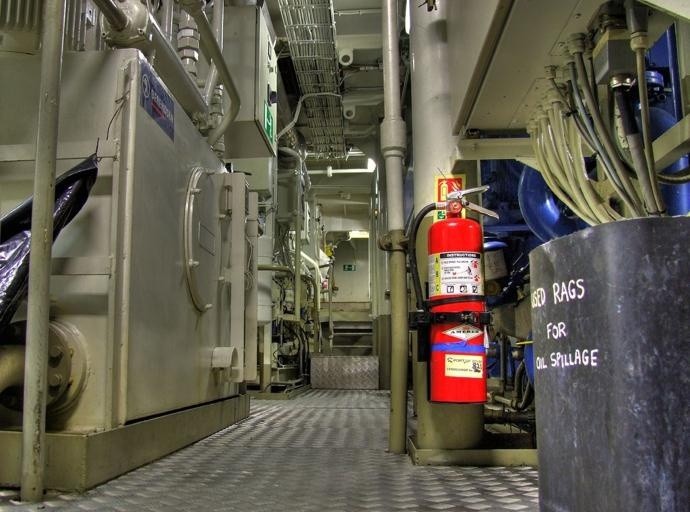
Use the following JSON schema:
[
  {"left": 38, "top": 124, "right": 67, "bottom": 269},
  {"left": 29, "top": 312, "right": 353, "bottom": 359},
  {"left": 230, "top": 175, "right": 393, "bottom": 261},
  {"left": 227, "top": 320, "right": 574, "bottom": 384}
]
[{"left": 407, "top": 185, "right": 500, "bottom": 402}]
